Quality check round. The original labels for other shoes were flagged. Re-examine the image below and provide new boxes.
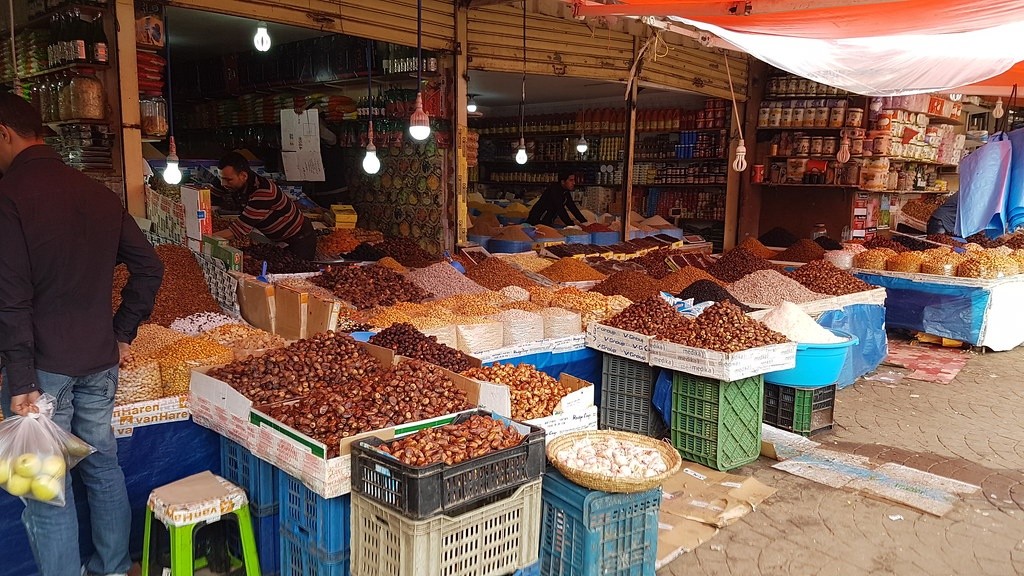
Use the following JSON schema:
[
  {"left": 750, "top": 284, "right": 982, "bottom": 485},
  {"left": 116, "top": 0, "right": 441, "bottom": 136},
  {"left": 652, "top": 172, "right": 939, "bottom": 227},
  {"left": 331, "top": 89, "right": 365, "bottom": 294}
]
[{"left": 81, "top": 564, "right": 128, "bottom": 576}]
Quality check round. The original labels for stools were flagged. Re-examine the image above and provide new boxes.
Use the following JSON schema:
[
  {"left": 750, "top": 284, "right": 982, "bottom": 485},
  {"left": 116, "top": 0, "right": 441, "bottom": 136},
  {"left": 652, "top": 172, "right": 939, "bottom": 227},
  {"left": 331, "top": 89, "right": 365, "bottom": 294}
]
[{"left": 141, "top": 470, "right": 262, "bottom": 576}]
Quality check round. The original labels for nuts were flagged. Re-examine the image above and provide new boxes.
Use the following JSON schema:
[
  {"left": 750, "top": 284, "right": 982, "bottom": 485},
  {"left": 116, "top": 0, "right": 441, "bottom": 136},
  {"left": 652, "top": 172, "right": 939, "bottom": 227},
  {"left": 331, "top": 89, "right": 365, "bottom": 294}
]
[
  {"left": 198, "top": 322, "right": 573, "bottom": 466},
  {"left": 596, "top": 292, "right": 792, "bottom": 354}
]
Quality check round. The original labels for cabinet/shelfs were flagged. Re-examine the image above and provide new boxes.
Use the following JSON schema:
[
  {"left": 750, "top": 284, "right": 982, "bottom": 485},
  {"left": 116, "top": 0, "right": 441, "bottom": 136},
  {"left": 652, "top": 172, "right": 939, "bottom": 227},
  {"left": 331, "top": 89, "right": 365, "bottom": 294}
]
[
  {"left": 0, "top": 0, "right": 456, "bottom": 260},
  {"left": 466, "top": 60, "right": 976, "bottom": 256}
]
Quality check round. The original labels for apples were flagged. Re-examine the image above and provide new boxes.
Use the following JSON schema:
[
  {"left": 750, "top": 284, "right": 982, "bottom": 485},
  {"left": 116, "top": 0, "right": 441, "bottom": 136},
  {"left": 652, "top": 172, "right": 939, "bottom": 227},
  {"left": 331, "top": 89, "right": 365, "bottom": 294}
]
[{"left": 0, "top": 452, "right": 66, "bottom": 500}]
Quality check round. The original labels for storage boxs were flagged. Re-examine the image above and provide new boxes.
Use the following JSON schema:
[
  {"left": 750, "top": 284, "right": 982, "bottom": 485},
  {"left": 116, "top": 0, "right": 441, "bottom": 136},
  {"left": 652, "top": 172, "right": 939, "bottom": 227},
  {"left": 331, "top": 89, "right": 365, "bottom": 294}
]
[{"left": 221, "top": 315, "right": 837, "bottom": 576}]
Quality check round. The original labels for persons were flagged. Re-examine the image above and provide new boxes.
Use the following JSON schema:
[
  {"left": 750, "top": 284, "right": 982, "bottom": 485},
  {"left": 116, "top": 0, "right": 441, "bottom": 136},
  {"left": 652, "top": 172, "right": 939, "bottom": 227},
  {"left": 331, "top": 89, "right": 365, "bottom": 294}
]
[
  {"left": 925, "top": 190, "right": 959, "bottom": 234},
  {"left": 0, "top": 93, "right": 164, "bottom": 576},
  {"left": 522, "top": 166, "right": 591, "bottom": 228},
  {"left": 210, "top": 150, "right": 317, "bottom": 259}
]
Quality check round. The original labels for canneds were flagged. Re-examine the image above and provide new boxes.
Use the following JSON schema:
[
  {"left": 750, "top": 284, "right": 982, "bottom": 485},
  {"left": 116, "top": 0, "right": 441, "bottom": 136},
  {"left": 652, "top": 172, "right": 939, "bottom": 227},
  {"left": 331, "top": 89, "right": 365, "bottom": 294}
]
[
  {"left": 491, "top": 129, "right": 727, "bottom": 221},
  {"left": 749, "top": 75, "right": 865, "bottom": 185}
]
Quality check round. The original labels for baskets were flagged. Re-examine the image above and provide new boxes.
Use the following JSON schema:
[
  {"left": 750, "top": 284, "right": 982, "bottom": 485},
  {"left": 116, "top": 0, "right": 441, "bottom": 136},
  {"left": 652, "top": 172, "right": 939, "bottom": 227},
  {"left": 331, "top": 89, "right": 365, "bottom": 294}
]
[
  {"left": 547, "top": 430, "right": 683, "bottom": 493},
  {"left": 350, "top": 412, "right": 546, "bottom": 522}
]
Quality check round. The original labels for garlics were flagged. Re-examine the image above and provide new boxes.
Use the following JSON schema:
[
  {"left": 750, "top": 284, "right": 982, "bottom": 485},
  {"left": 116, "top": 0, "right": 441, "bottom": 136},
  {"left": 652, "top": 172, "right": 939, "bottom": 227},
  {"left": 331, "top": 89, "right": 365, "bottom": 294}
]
[{"left": 556, "top": 436, "right": 668, "bottom": 478}]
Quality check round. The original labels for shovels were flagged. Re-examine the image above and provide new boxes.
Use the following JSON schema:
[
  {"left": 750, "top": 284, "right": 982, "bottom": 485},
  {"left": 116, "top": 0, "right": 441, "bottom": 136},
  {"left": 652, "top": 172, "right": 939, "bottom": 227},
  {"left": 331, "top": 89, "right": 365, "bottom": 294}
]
[
  {"left": 443, "top": 250, "right": 465, "bottom": 274},
  {"left": 256, "top": 261, "right": 269, "bottom": 284}
]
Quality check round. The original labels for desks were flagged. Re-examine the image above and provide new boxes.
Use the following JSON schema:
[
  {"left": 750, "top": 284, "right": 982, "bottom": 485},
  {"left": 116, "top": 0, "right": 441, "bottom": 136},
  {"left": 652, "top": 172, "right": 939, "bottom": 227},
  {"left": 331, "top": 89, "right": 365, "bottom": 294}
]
[
  {"left": 0, "top": 415, "right": 220, "bottom": 576},
  {"left": 710, "top": 246, "right": 1024, "bottom": 354}
]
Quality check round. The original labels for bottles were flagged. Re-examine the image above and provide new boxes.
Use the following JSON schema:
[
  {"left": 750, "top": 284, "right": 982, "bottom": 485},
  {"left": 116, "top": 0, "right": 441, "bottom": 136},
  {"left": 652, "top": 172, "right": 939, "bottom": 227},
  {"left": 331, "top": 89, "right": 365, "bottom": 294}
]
[
  {"left": 680, "top": 110, "right": 696, "bottom": 130},
  {"left": 383, "top": 42, "right": 437, "bottom": 73},
  {"left": 490, "top": 173, "right": 558, "bottom": 182},
  {"left": 537, "top": 137, "right": 625, "bottom": 160},
  {"left": 696, "top": 133, "right": 719, "bottom": 157},
  {"left": 471, "top": 112, "right": 576, "bottom": 134},
  {"left": 31, "top": 68, "right": 105, "bottom": 119},
  {"left": 356, "top": 96, "right": 386, "bottom": 119},
  {"left": 656, "top": 161, "right": 728, "bottom": 184},
  {"left": 635, "top": 134, "right": 677, "bottom": 158},
  {"left": 675, "top": 130, "right": 700, "bottom": 158},
  {"left": 140, "top": 98, "right": 168, "bottom": 136},
  {"left": 576, "top": 107, "right": 679, "bottom": 134},
  {"left": 28, "top": 0, "right": 109, "bottom": 68},
  {"left": 810, "top": 224, "right": 827, "bottom": 239}
]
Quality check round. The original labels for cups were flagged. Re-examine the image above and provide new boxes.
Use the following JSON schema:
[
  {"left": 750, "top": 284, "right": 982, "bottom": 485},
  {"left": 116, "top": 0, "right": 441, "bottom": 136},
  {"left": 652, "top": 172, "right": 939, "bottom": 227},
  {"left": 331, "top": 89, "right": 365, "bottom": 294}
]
[
  {"left": 696, "top": 97, "right": 731, "bottom": 129},
  {"left": 764, "top": 76, "right": 848, "bottom": 94}
]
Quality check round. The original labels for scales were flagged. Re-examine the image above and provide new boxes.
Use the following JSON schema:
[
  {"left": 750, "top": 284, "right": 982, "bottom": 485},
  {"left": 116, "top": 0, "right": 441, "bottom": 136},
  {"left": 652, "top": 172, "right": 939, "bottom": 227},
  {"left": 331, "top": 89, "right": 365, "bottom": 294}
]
[
  {"left": 669, "top": 208, "right": 706, "bottom": 244},
  {"left": 484, "top": 197, "right": 510, "bottom": 207}
]
